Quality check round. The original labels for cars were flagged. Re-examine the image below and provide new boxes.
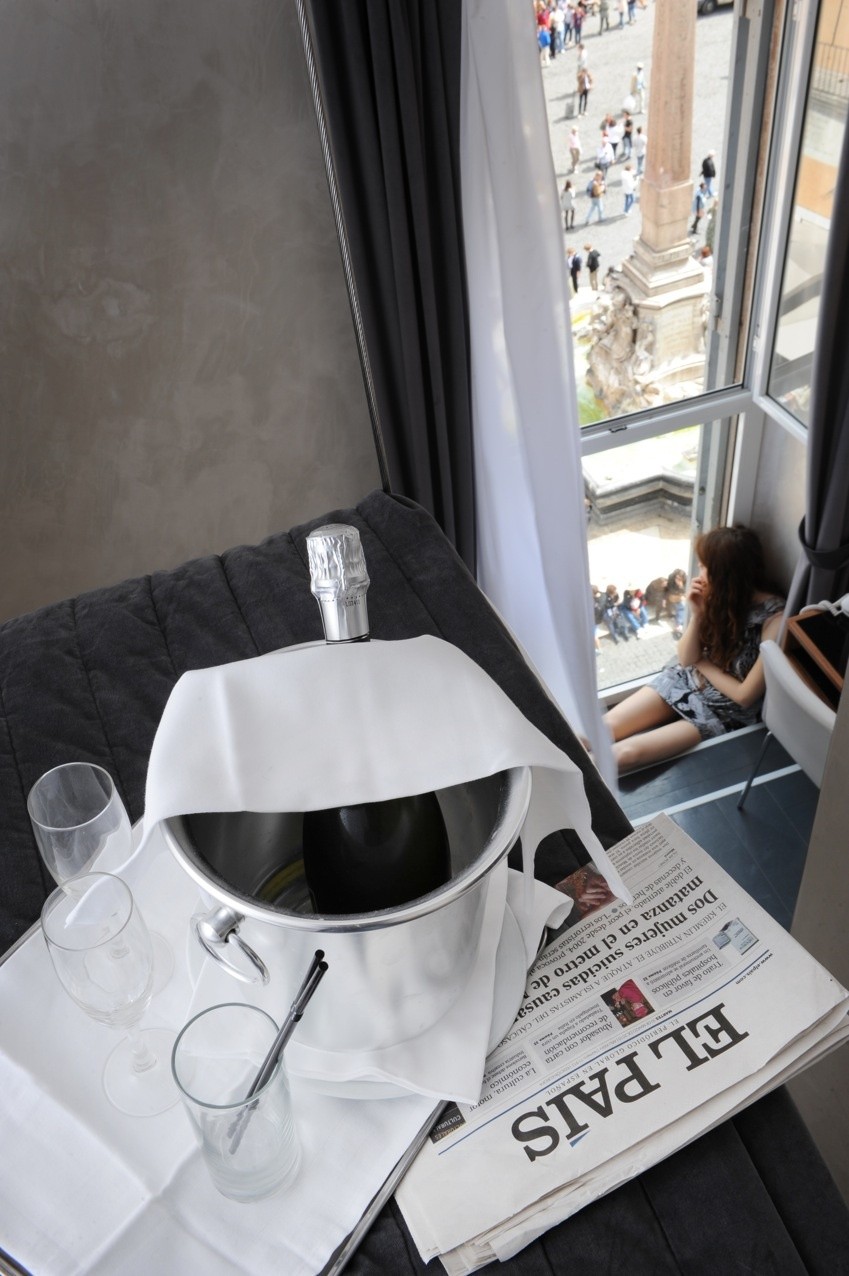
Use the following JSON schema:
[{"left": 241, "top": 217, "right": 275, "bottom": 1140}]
[{"left": 698, "top": 0, "right": 734, "bottom": 15}]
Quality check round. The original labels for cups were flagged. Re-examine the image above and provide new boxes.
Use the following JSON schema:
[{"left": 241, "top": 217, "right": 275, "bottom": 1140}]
[
  {"left": 170, "top": 1002, "right": 303, "bottom": 1204},
  {"left": 27, "top": 762, "right": 137, "bottom": 899}
]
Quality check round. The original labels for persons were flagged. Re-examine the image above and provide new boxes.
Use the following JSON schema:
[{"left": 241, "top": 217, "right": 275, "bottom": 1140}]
[
  {"left": 591, "top": 584, "right": 650, "bottom": 655},
  {"left": 603, "top": 978, "right": 648, "bottom": 1023},
  {"left": 602, "top": 522, "right": 785, "bottom": 772},
  {"left": 647, "top": 568, "right": 687, "bottom": 640}
]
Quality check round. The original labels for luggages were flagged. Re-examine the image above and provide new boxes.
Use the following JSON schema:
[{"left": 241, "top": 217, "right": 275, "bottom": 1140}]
[{"left": 565, "top": 91, "right": 575, "bottom": 119}]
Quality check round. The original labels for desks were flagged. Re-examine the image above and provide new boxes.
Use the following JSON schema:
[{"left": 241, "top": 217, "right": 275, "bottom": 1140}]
[
  {"left": 0, "top": 489, "right": 849, "bottom": 1276},
  {"left": 781, "top": 607, "right": 849, "bottom": 691}
]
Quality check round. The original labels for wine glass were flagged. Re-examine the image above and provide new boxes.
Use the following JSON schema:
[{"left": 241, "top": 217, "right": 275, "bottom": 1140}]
[{"left": 39, "top": 870, "right": 179, "bottom": 1118}]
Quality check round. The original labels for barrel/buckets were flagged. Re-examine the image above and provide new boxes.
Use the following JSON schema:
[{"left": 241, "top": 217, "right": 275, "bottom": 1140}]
[{"left": 160, "top": 766, "right": 534, "bottom": 1052}]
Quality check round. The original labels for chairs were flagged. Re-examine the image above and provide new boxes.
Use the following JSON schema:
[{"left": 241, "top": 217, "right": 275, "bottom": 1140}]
[{"left": 738, "top": 640, "right": 837, "bottom": 812}]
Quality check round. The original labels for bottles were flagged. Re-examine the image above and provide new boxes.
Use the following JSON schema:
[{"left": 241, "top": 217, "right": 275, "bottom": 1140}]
[{"left": 303, "top": 522, "right": 454, "bottom": 915}]
